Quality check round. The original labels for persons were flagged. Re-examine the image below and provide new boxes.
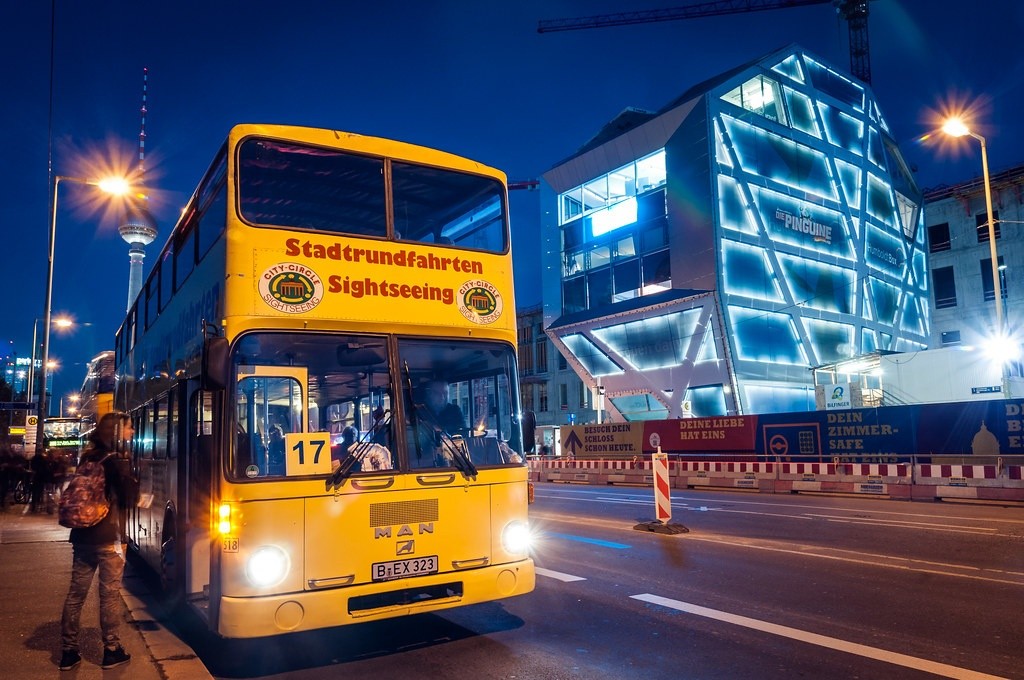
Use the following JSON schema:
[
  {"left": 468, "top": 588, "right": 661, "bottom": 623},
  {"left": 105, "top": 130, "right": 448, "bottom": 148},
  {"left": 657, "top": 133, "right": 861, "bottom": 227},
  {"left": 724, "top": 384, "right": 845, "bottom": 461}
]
[
  {"left": 29, "top": 445, "right": 71, "bottom": 514},
  {"left": 267, "top": 424, "right": 286, "bottom": 474},
  {"left": 337, "top": 425, "right": 363, "bottom": 473},
  {"left": 0, "top": 435, "right": 23, "bottom": 506},
  {"left": 414, "top": 377, "right": 469, "bottom": 440},
  {"left": 58, "top": 410, "right": 140, "bottom": 672}
]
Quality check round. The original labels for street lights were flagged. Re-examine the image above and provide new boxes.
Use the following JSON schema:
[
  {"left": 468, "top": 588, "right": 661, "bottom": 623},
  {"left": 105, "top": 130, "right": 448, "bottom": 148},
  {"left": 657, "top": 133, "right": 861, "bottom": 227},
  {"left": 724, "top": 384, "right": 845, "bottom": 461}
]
[
  {"left": 28, "top": 175, "right": 132, "bottom": 515},
  {"left": 941, "top": 117, "right": 1007, "bottom": 340},
  {"left": 25, "top": 314, "right": 74, "bottom": 420}
]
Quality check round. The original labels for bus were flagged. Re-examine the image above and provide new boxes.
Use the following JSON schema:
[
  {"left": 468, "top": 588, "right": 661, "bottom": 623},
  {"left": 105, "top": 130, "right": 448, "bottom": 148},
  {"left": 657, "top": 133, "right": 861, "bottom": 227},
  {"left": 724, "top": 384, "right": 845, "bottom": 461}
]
[{"left": 112, "top": 124, "right": 536, "bottom": 639}]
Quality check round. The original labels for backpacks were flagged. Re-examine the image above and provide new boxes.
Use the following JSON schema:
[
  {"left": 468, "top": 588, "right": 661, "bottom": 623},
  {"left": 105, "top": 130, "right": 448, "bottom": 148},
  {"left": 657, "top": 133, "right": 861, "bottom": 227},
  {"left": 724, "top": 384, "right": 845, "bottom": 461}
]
[{"left": 58, "top": 452, "right": 119, "bottom": 528}]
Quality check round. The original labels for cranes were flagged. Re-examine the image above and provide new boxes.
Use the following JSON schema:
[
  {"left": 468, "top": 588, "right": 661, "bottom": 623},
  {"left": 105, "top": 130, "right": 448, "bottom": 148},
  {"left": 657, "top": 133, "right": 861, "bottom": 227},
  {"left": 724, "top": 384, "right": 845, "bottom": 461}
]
[{"left": 536, "top": 0, "right": 872, "bottom": 83}]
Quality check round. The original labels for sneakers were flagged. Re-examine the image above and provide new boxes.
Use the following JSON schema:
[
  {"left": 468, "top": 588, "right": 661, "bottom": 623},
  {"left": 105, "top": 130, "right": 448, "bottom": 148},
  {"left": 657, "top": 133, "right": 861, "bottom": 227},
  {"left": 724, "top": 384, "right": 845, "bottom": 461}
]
[
  {"left": 60, "top": 649, "right": 81, "bottom": 670},
  {"left": 101, "top": 642, "right": 131, "bottom": 669}
]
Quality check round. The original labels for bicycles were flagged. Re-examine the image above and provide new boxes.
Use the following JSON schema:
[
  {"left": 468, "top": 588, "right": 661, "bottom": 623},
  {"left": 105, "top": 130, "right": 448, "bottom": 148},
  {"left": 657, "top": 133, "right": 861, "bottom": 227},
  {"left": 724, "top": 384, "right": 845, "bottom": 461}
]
[{"left": 14, "top": 470, "right": 33, "bottom": 504}]
